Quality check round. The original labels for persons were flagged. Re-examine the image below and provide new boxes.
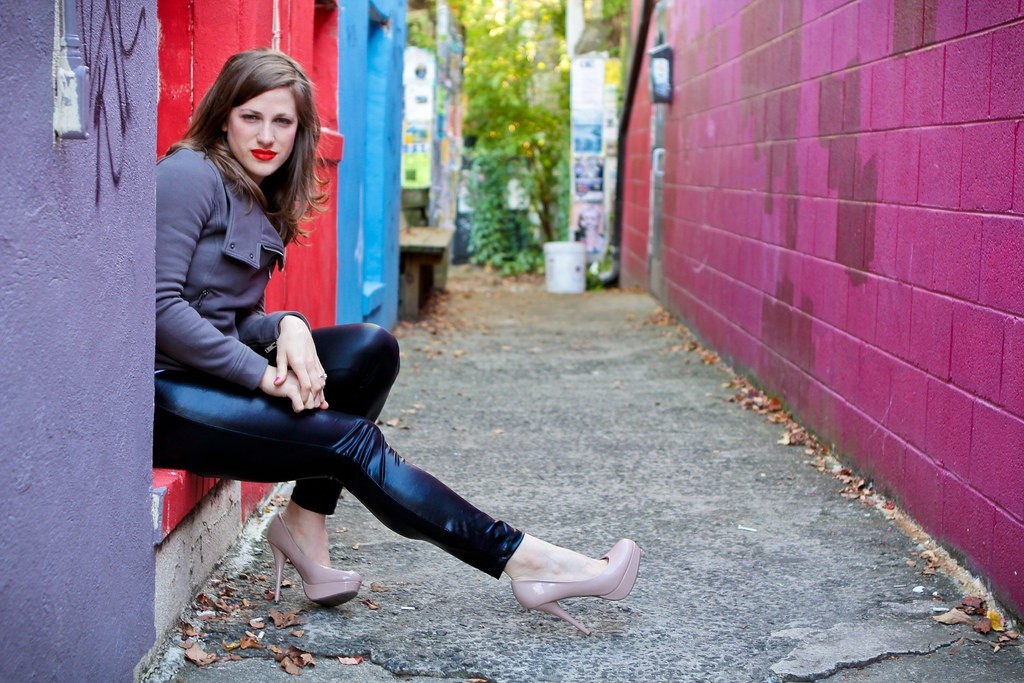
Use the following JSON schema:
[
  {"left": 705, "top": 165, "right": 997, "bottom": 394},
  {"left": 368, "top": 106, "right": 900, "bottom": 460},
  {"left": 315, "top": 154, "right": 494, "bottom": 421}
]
[{"left": 156, "top": 44, "right": 644, "bottom": 642}]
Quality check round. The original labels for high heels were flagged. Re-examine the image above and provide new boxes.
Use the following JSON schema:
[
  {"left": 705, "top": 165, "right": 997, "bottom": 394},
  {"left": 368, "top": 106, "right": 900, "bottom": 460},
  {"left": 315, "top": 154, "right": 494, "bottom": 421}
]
[
  {"left": 511, "top": 539, "right": 640, "bottom": 636},
  {"left": 267, "top": 512, "right": 361, "bottom": 606}
]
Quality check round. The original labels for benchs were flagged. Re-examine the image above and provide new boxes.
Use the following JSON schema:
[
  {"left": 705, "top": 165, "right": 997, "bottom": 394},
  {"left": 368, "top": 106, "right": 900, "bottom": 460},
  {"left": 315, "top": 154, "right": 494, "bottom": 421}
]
[{"left": 399, "top": 211, "right": 453, "bottom": 318}]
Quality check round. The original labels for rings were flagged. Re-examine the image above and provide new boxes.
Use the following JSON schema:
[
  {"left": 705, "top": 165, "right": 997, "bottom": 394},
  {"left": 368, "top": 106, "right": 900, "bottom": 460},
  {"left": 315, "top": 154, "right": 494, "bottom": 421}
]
[{"left": 318, "top": 373, "right": 328, "bottom": 381}]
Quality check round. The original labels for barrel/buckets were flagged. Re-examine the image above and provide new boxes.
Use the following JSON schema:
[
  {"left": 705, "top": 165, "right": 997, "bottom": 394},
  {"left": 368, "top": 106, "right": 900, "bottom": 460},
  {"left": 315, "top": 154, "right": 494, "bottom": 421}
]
[{"left": 543, "top": 240, "right": 587, "bottom": 294}]
